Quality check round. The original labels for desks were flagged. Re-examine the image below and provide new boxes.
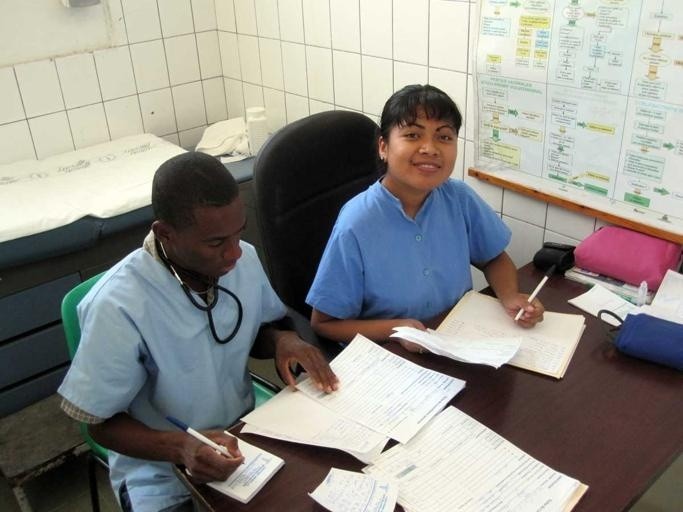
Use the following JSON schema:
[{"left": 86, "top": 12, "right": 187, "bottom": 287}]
[{"left": 173, "top": 264, "right": 683, "bottom": 511}]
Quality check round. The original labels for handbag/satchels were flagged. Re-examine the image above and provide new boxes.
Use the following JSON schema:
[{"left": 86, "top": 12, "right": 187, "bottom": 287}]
[{"left": 571, "top": 223, "right": 683, "bottom": 293}]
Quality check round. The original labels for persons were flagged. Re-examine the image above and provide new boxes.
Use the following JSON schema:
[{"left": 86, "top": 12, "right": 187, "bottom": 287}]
[
  {"left": 302, "top": 82, "right": 544, "bottom": 357},
  {"left": 56, "top": 149, "right": 341, "bottom": 511}
]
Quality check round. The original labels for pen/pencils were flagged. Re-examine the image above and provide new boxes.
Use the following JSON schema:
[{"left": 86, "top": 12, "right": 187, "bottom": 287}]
[
  {"left": 164, "top": 415, "right": 246, "bottom": 465},
  {"left": 514, "top": 263, "right": 557, "bottom": 321}
]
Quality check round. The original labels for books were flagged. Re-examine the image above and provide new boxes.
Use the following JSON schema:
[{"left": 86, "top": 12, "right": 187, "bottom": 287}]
[
  {"left": 182, "top": 427, "right": 287, "bottom": 503},
  {"left": 422, "top": 285, "right": 588, "bottom": 382}
]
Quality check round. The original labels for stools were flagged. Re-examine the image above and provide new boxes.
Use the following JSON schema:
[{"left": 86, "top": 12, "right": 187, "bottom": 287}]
[{"left": 1, "top": 393, "right": 100, "bottom": 511}]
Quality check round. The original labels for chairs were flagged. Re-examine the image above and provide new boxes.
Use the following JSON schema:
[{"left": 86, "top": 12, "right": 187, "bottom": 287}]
[
  {"left": 254, "top": 112, "right": 387, "bottom": 384},
  {"left": 62, "top": 259, "right": 284, "bottom": 507}
]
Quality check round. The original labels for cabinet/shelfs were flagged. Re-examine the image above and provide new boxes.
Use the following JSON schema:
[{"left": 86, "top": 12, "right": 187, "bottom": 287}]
[{"left": 1, "top": 245, "right": 109, "bottom": 419}]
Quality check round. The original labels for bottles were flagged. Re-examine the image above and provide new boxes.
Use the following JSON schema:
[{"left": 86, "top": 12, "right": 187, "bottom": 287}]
[{"left": 247, "top": 106, "right": 268, "bottom": 157}]
